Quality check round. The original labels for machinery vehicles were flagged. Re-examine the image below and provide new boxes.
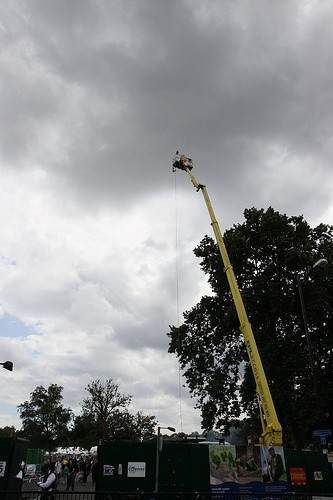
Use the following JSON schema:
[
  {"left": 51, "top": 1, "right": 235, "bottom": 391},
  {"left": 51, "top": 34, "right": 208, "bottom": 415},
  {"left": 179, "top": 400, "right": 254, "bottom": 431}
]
[{"left": 170, "top": 151, "right": 283, "bottom": 444}]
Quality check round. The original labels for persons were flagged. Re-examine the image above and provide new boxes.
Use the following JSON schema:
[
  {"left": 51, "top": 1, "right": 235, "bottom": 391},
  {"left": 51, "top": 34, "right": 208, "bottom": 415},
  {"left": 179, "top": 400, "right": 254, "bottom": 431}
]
[{"left": 20, "top": 434, "right": 333, "bottom": 500}]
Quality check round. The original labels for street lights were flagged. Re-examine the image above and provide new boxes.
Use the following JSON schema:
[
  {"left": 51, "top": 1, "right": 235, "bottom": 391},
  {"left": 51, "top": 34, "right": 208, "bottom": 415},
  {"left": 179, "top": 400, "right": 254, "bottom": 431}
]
[{"left": 154, "top": 426, "right": 176, "bottom": 492}]
